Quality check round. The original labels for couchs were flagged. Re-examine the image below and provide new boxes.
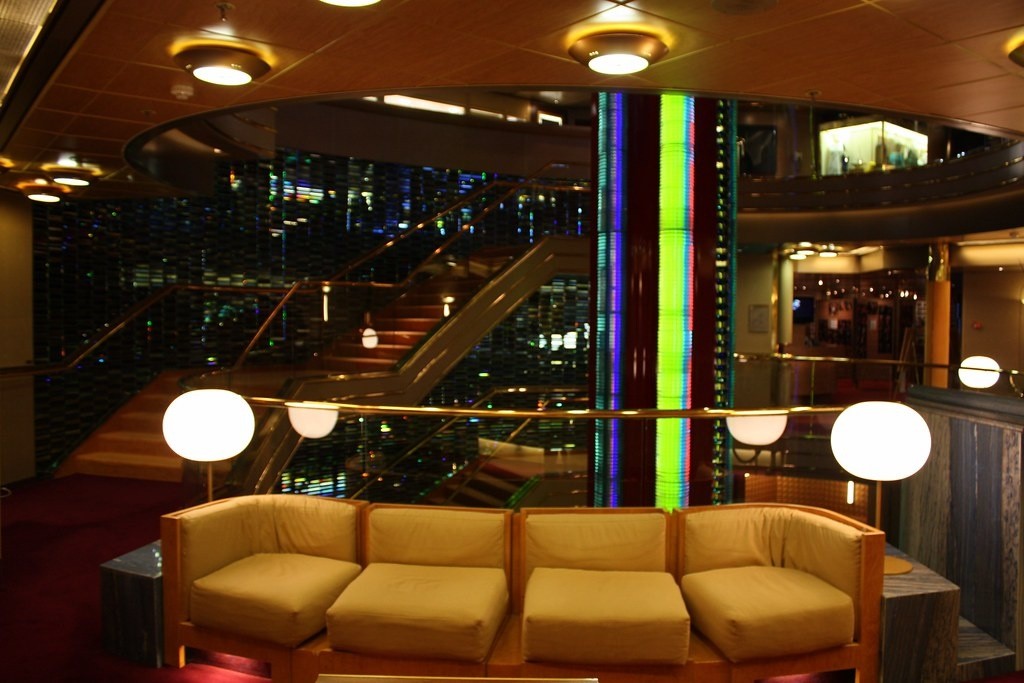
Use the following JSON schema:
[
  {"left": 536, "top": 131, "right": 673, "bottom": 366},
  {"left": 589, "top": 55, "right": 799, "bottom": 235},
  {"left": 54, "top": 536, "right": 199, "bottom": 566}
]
[{"left": 160, "top": 494, "right": 886, "bottom": 683}]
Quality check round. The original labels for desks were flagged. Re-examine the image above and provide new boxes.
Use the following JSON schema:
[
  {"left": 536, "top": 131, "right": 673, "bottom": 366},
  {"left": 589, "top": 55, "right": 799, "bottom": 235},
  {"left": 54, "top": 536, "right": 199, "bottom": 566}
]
[
  {"left": 880, "top": 543, "right": 958, "bottom": 683},
  {"left": 100, "top": 539, "right": 163, "bottom": 668}
]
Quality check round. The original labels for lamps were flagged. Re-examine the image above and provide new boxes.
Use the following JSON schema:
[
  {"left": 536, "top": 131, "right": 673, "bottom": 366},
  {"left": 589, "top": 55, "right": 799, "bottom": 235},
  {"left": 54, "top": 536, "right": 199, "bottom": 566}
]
[
  {"left": 25, "top": 186, "right": 61, "bottom": 203},
  {"left": 54, "top": 172, "right": 92, "bottom": 186},
  {"left": 173, "top": 47, "right": 271, "bottom": 86},
  {"left": 568, "top": 31, "right": 668, "bottom": 74},
  {"left": 831, "top": 401, "right": 931, "bottom": 574},
  {"left": 724, "top": 415, "right": 788, "bottom": 470},
  {"left": 162, "top": 389, "right": 255, "bottom": 502}
]
